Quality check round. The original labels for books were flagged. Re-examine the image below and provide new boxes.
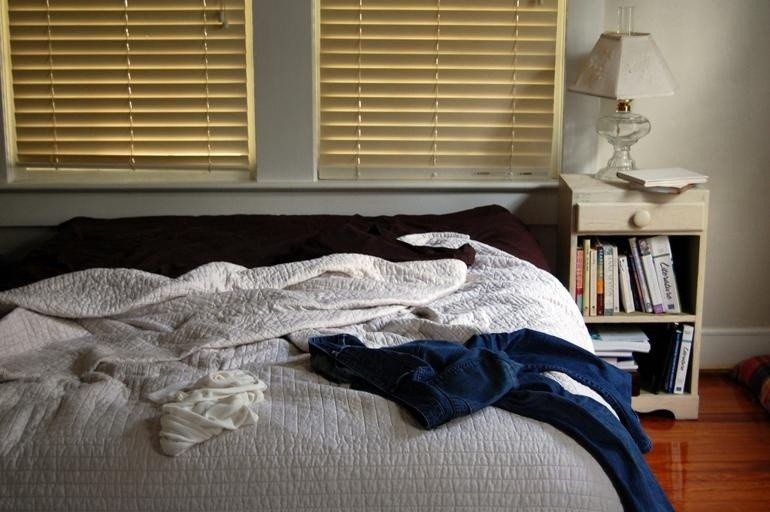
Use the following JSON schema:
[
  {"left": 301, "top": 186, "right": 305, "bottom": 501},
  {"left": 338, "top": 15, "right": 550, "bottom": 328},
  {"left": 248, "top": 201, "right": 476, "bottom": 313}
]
[
  {"left": 577, "top": 235, "right": 699, "bottom": 394},
  {"left": 615, "top": 164, "right": 709, "bottom": 195}
]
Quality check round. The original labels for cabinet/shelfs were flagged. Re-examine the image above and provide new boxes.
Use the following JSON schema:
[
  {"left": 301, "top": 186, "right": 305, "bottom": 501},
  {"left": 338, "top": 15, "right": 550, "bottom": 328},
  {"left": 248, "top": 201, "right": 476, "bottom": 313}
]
[{"left": 561, "top": 174, "right": 710, "bottom": 420}]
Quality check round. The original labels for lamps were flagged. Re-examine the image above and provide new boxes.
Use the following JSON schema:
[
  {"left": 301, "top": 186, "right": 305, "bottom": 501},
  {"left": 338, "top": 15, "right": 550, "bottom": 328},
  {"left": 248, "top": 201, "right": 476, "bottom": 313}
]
[{"left": 569, "top": 6, "right": 680, "bottom": 180}]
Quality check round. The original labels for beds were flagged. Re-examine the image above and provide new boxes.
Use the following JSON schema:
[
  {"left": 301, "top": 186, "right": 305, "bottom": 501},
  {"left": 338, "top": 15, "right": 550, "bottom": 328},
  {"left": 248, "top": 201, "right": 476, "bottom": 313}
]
[{"left": 0, "top": 204, "right": 626, "bottom": 512}]
